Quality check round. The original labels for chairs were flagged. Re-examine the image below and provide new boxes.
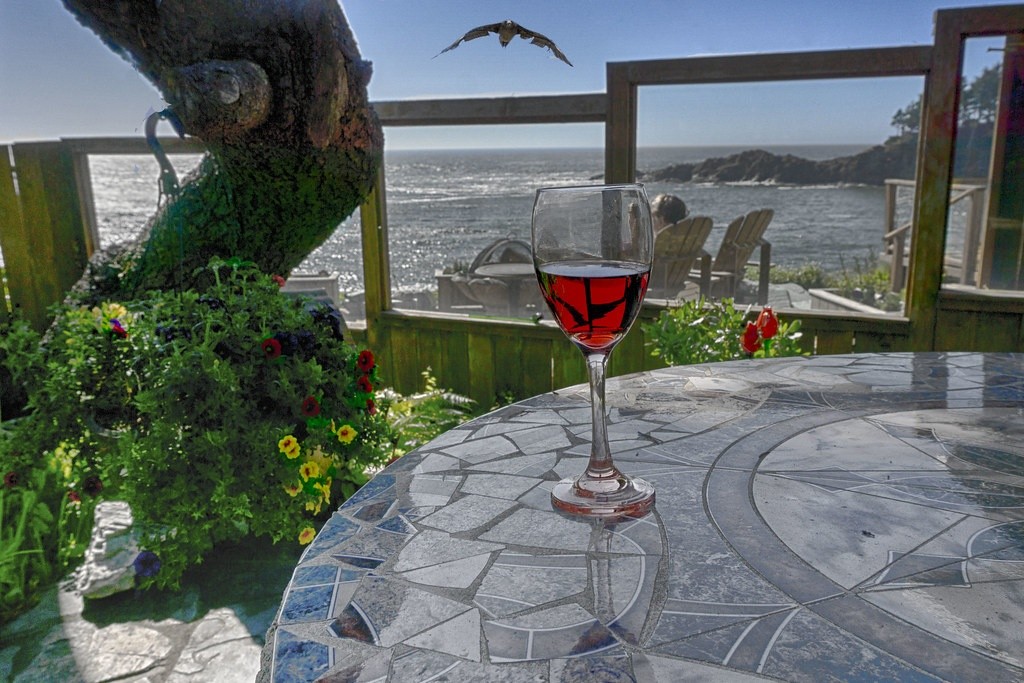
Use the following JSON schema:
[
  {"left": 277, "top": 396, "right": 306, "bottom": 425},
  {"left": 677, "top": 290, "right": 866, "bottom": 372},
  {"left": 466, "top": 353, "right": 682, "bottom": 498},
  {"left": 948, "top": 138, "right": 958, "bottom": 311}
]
[{"left": 541, "top": 208, "right": 774, "bottom": 307}]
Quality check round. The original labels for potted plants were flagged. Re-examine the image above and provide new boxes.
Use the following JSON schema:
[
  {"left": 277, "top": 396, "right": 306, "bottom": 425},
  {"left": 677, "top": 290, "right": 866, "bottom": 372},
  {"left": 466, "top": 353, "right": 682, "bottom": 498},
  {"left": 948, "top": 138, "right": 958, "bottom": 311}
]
[{"left": 434, "top": 260, "right": 485, "bottom": 313}]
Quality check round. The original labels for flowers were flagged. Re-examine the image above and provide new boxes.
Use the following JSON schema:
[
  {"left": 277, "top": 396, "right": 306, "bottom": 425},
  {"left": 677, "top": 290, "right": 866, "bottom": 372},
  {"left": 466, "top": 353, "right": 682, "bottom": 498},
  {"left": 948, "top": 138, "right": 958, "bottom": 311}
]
[
  {"left": 0, "top": 109, "right": 414, "bottom": 592},
  {"left": 738, "top": 306, "right": 780, "bottom": 358}
]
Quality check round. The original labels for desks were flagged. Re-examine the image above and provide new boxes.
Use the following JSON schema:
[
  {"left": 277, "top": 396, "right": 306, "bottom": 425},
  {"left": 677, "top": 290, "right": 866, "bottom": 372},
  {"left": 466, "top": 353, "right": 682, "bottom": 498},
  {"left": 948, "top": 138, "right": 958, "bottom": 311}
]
[
  {"left": 474, "top": 264, "right": 537, "bottom": 316},
  {"left": 254, "top": 352, "right": 1024, "bottom": 682}
]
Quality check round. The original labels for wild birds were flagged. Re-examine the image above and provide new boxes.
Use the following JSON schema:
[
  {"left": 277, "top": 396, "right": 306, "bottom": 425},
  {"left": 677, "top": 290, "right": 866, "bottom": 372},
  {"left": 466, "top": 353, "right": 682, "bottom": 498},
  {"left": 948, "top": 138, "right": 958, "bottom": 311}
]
[{"left": 433, "top": 20, "right": 573, "bottom": 69}]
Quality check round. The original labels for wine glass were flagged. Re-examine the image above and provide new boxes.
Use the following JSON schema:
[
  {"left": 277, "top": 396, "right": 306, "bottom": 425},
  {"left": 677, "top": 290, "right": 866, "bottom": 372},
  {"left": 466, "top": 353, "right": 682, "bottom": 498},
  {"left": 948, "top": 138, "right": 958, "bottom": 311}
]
[{"left": 531, "top": 183, "right": 656, "bottom": 518}]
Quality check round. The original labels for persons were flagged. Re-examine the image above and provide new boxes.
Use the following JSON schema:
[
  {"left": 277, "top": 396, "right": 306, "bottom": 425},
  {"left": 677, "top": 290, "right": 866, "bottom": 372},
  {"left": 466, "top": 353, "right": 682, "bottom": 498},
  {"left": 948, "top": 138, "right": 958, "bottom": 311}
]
[{"left": 650, "top": 192, "right": 687, "bottom": 233}]
